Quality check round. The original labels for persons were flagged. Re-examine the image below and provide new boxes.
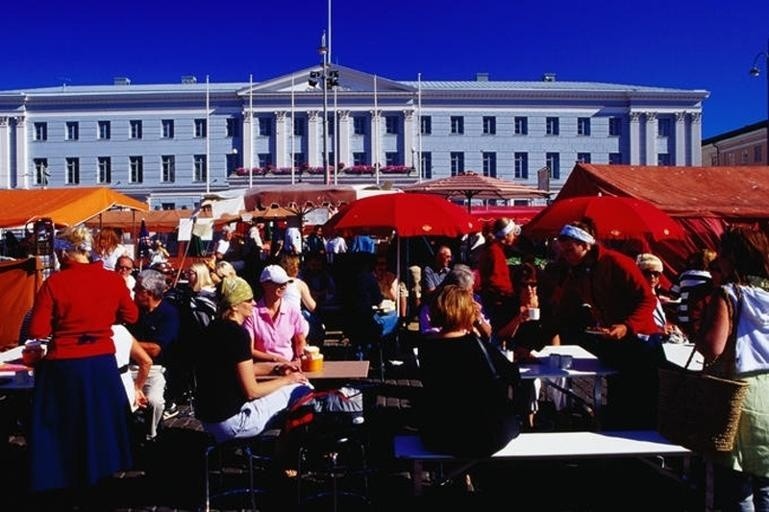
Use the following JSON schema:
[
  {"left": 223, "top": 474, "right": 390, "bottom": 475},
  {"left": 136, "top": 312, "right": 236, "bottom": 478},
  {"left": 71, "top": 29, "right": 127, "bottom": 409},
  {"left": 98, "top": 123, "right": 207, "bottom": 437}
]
[{"left": 2, "top": 218, "right": 769, "bottom": 512}]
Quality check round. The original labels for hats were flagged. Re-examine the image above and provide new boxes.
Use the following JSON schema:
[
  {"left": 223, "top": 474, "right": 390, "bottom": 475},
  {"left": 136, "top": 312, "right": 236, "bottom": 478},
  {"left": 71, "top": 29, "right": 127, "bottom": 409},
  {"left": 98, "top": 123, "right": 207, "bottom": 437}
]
[
  {"left": 635, "top": 251, "right": 663, "bottom": 273},
  {"left": 258, "top": 264, "right": 295, "bottom": 285}
]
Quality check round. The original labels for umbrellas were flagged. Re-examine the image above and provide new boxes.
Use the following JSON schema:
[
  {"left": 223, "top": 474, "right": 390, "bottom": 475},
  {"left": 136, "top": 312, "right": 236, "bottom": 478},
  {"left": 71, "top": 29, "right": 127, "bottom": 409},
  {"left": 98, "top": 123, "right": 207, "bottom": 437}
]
[
  {"left": 322, "top": 193, "right": 482, "bottom": 318},
  {"left": 524, "top": 192, "right": 686, "bottom": 244}
]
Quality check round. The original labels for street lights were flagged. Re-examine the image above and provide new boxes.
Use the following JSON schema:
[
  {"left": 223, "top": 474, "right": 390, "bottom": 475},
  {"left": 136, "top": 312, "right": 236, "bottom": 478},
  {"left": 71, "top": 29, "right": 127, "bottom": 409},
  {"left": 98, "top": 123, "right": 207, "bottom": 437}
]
[
  {"left": 750, "top": 50, "right": 769, "bottom": 82},
  {"left": 308, "top": 28, "right": 343, "bottom": 190}
]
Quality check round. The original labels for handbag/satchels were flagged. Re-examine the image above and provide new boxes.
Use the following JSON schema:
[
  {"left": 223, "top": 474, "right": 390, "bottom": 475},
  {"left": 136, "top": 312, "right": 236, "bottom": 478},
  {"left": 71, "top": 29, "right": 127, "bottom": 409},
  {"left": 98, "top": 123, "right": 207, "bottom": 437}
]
[{"left": 650, "top": 278, "right": 749, "bottom": 457}]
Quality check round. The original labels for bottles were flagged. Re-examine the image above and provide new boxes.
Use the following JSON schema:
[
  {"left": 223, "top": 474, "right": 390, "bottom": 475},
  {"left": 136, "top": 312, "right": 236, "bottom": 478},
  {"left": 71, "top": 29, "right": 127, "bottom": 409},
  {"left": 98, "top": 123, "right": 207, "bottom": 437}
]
[{"left": 21, "top": 340, "right": 45, "bottom": 365}]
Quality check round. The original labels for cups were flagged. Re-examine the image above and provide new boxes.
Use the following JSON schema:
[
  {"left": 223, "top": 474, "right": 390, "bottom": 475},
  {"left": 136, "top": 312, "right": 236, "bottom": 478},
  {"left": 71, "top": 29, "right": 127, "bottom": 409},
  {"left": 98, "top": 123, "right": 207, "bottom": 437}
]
[
  {"left": 500, "top": 350, "right": 515, "bottom": 363},
  {"left": 301, "top": 353, "right": 324, "bottom": 374},
  {"left": 529, "top": 308, "right": 540, "bottom": 320}
]
